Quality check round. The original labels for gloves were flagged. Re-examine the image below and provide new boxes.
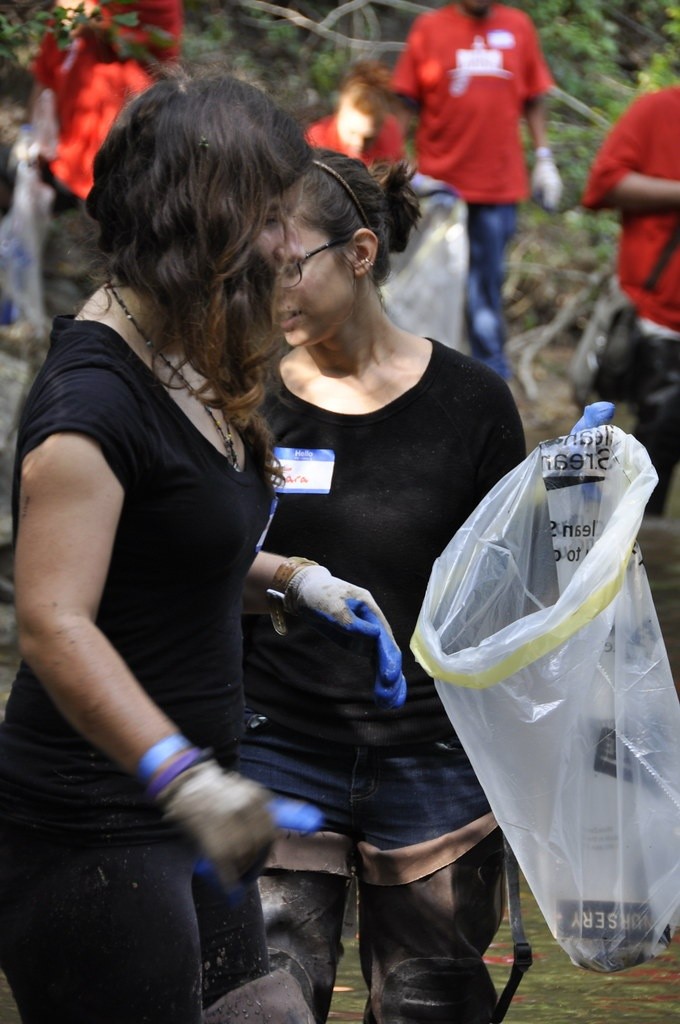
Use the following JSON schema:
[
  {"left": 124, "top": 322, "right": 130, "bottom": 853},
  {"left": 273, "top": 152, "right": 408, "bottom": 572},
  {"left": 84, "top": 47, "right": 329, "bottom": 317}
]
[
  {"left": 152, "top": 758, "right": 326, "bottom": 916},
  {"left": 283, "top": 565, "right": 407, "bottom": 712},
  {"left": 565, "top": 400, "right": 616, "bottom": 507},
  {"left": 529, "top": 157, "right": 564, "bottom": 213}
]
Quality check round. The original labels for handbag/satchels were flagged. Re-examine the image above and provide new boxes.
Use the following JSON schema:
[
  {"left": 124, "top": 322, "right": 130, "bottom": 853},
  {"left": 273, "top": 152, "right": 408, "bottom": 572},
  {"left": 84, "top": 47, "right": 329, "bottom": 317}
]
[
  {"left": 408, "top": 423, "right": 680, "bottom": 973},
  {"left": 565, "top": 274, "right": 636, "bottom": 414}
]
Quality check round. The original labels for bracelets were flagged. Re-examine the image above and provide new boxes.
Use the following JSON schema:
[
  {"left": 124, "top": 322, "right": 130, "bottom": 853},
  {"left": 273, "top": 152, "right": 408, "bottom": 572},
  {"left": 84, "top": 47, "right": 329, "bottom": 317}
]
[
  {"left": 267, "top": 557, "right": 317, "bottom": 635},
  {"left": 137, "top": 733, "right": 192, "bottom": 783}
]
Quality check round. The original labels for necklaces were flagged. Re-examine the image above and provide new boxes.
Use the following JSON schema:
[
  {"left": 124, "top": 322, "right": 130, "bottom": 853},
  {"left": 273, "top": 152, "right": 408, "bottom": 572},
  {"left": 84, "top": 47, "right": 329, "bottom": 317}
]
[{"left": 107, "top": 279, "right": 241, "bottom": 473}]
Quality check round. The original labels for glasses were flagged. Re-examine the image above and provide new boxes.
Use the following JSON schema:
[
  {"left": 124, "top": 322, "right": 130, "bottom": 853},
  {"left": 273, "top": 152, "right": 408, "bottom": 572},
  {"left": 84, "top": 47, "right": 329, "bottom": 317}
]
[{"left": 273, "top": 234, "right": 354, "bottom": 288}]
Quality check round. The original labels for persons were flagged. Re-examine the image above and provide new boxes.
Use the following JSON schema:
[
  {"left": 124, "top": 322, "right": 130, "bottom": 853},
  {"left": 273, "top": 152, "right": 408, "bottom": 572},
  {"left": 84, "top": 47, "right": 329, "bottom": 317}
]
[
  {"left": 33, "top": 0, "right": 183, "bottom": 321},
  {"left": 583, "top": 85, "right": 679, "bottom": 517},
  {"left": 390, "top": 0, "right": 561, "bottom": 379},
  {"left": 241, "top": 149, "right": 615, "bottom": 1024},
  {"left": 0, "top": 75, "right": 407, "bottom": 1024},
  {"left": 304, "top": 64, "right": 406, "bottom": 168}
]
